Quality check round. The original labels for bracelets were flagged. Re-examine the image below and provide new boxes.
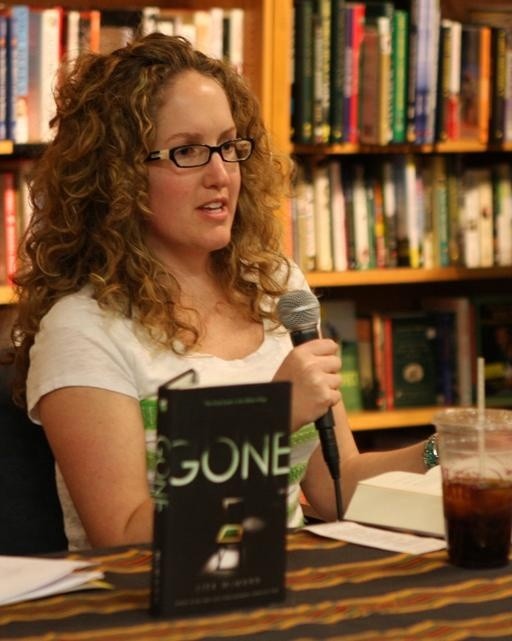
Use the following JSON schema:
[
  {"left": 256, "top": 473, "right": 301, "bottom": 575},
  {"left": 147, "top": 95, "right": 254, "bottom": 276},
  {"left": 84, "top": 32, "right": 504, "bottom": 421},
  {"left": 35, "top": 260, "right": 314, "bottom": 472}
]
[{"left": 424, "top": 433, "right": 438, "bottom": 469}]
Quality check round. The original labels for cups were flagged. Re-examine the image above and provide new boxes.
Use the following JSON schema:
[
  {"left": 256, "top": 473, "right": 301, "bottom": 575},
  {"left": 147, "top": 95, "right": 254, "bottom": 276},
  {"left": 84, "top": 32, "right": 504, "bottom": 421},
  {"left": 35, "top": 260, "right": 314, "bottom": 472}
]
[{"left": 431, "top": 407, "right": 512, "bottom": 568}]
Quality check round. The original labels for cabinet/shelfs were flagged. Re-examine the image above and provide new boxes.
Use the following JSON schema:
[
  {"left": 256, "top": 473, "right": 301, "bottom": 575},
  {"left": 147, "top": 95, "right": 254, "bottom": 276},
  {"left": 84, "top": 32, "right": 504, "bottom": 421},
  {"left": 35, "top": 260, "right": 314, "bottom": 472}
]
[{"left": 0, "top": 0, "right": 512, "bottom": 531}]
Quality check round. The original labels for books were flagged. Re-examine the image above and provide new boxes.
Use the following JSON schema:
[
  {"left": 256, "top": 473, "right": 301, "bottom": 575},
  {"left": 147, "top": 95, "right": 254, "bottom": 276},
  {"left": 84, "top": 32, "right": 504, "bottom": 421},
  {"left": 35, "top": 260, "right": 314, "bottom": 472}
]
[
  {"left": 139, "top": 7, "right": 244, "bottom": 76},
  {"left": 0, "top": 5, "right": 100, "bottom": 145},
  {"left": 289, "top": 151, "right": 512, "bottom": 272},
  {"left": 291, "top": 0, "right": 512, "bottom": 151},
  {"left": 342, "top": 461, "right": 451, "bottom": 544},
  {"left": 316, "top": 296, "right": 512, "bottom": 413},
  {"left": 0, "top": 170, "right": 35, "bottom": 306},
  {"left": 143, "top": 369, "right": 294, "bottom": 623}
]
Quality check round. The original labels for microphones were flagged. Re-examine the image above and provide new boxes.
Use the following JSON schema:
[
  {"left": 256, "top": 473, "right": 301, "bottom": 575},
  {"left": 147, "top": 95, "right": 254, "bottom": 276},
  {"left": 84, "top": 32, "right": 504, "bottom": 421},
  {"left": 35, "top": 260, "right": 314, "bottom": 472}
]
[{"left": 277, "top": 290, "right": 341, "bottom": 480}]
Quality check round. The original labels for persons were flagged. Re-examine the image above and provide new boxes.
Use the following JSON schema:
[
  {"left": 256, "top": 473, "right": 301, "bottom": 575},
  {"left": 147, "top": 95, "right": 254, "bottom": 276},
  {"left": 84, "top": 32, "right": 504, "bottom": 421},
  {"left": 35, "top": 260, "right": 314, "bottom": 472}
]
[{"left": 8, "top": 32, "right": 436, "bottom": 551}]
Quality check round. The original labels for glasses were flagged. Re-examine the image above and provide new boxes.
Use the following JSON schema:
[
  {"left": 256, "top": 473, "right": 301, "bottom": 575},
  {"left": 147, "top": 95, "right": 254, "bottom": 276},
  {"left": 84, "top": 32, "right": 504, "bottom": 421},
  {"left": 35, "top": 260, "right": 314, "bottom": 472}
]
[{"left": 144, "top": 137, "right": 255, "bottom": 168}]
